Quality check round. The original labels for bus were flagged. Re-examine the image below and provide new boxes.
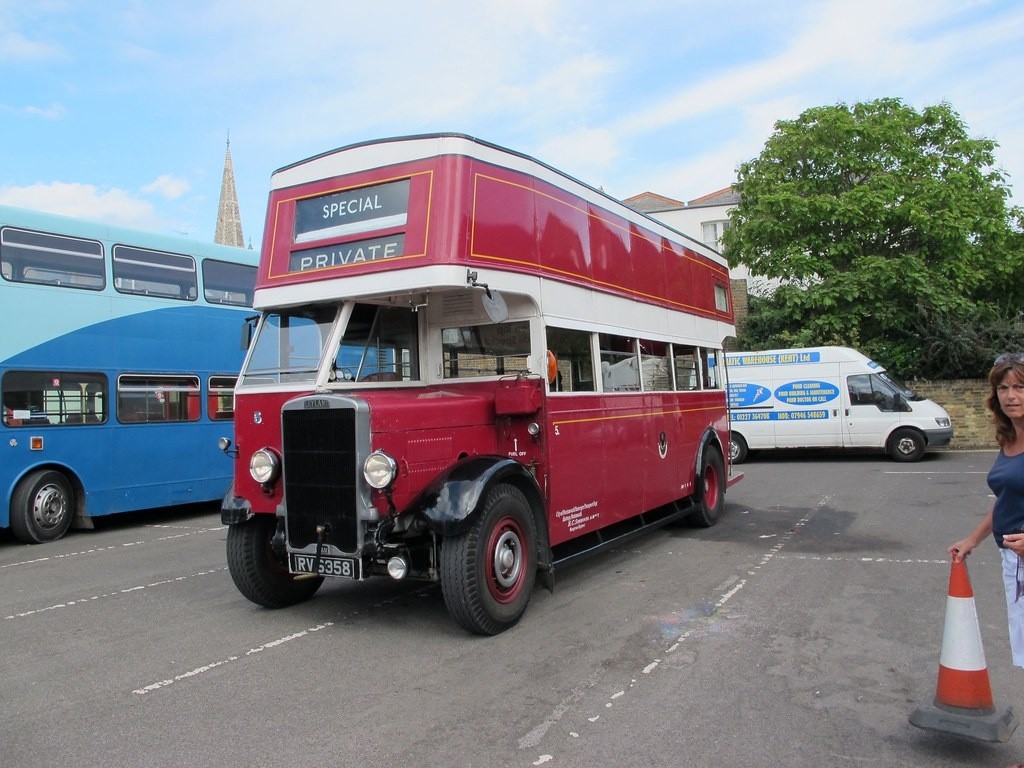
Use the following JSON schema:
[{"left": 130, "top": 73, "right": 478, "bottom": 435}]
[
  {"left": 218, "top": 130, "right": 747, "bottom": 636},
  {"left": 0, "top": 205, "right": 261, "bottom": 546}
]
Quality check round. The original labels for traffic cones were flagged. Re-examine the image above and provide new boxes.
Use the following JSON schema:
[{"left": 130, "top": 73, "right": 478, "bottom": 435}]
[{"left": 908, "top": 546, "right": 1021, "bottom": 744}]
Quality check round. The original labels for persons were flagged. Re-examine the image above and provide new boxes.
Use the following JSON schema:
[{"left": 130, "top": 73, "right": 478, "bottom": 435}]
[{"left": 947, "top": 353, "right": 1024, "bottom": 669}]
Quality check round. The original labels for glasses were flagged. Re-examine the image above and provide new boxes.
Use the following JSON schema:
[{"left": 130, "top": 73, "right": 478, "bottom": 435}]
[{"left": 993, "top": 353, "right": 1024, "bottom": 366}]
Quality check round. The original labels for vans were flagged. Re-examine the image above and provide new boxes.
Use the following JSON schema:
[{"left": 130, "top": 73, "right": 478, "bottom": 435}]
[{"left": 688, "top": 345, "right": 953, "bottom": 462}]
